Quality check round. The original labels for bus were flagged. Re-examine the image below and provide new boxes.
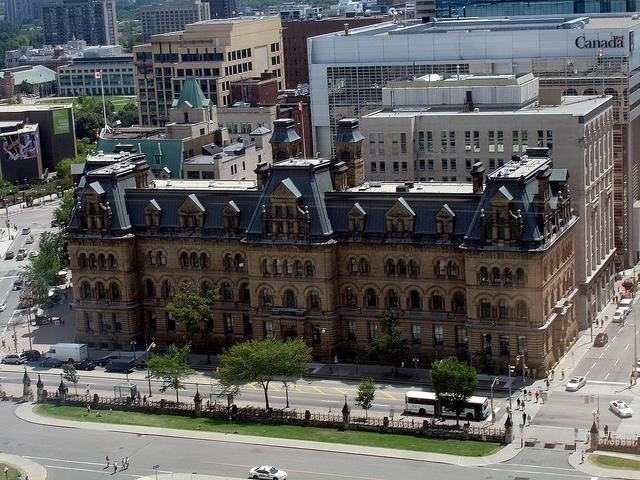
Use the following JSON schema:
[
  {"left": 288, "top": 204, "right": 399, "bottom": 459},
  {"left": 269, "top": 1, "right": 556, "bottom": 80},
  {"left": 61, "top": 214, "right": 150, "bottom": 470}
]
[{"left": 404, "top": 390, "right": 489, "bottom": 422}]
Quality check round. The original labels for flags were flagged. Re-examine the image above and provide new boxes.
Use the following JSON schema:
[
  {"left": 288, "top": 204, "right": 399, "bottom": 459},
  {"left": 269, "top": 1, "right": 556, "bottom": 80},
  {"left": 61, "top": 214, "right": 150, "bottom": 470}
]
[
  {"left": 297, "top": 101, "right": 303, "bottom": 112},
  {"left": 95, "top": 71, "right": 101, "bottom": 79}
]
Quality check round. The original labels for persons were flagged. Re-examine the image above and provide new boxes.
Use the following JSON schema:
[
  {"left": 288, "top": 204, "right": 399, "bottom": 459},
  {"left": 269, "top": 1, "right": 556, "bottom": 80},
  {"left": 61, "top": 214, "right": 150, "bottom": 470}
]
[
  {"left": 517, "top": 398, "right": 521, "bottom": 410},
  {"left": 604, "top": 425, "right": 609, "bottom": 437},
  {"left": 521, "top": 400, "right": 526, "bottom": 411},
  {"left": 612, "top": 296, "right": 615, "bottom": 303},
  {"left": 86, "top": 384, "right": 90, "bottom": 393},
  {"left": 551, "top": 370, "right": 554, "bottom": 378},
  {"left": 59, "top": 315, "right": 65, "bottom": 328},
  {"left": 595, "top": 319, "right": 599, "bottom": 326},
  {"left": 106, "top": 456, "right": 109, "bottom": 469},
  {"left": 125, "top": 457, "right": 129, "bottom": 469},
  {"left": 547, "top": 380, "right": 549, "bottom": 390},
  {"left": 523, "top": 389, "right": 528, "bottom": 400},
  {"left": 629, "top": 370, "right": 640, "bottom": 386},
  {"left": 522, "top": 412, "right": 526, "bottom": 425},
  {"left": 1, "top": 337, "right": 6, "bottom": 348},
  {"left": 528, "top": 390, "right": 532, "bottom": 400},
  {"left": 122, "top": 458, "right": 125, "bottom": 469},
  {"left": 601, "top": 319, "right": 603, "bottom": 327},
  {"left": 2, "top": 133, "right": 38, "bottom": 160},
  {"left": 527, "top": 414, "right": 531, "bottom": 426},
  {"left": 616, "top": 295, "right": 618, "bottom": 302},
  {"left": 16, "top": 475, "right": 22, "bottom": 480},
  {"left": 113, "top": 461, "right": 118, "bottom": 472},
  {"left": 539, "top": 389, "right": 549, "bottom": 404},
  {"left": 25, "top": 475, "right": 29, "bottom": 480},
  {"left": 585, "top": 431, "right": 590, "bottom": 441},
  {"left": 561, "top": 368, "right": 565, "bottom": 379},
  {"left": 534, "top": 390, "right": 539, "bottom": 403},
  {"left": 87, "top": 403, "right": 113, "bottom": 418}
]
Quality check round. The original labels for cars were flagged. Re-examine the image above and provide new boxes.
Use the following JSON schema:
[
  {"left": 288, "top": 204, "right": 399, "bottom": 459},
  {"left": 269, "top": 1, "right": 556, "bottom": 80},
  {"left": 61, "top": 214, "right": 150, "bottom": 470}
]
[
  {"left": 613, "top": 280, "right": 636, "bottom": 324},
  {"left": 632, "top": 361, "right": 640, "bottom": 377},
  {"left": 247, "top": 466, "right": 289, "bottom": 480},
  {"left": 1, "top": 226, "right": 38, "bottom": 320},
  {"left": 594, "top": 334, "right": 609, "bottom": 346},
  {"left": 609, "top": 400, "right": 633, "bottom": 418},
  {"left": 0, "top": 343, "right": 144, "bottom": 372},
  {"left": 566, "top": 376, "right": 587, "bottom": 392}
]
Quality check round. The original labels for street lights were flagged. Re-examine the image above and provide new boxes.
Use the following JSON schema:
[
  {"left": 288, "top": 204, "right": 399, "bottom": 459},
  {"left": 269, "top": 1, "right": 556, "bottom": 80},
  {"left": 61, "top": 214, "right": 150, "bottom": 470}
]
[
  {"left": 319, "top": 327, "right": 335, "bottom": 368},
  {"left": 130, "top": 340, "right": 138, "bottom": 361},
  {"left": 143, "top": 341, "right": 156, "bottom": 396},
  {"left": 412, "top": 357, "right": 420, "bottom": 379},
  {"left": 491, "top": 377, "right": 501, "bottom": 418},
  {"left": 515, "top": 354, "right": 527, "bottom": 388}
]
